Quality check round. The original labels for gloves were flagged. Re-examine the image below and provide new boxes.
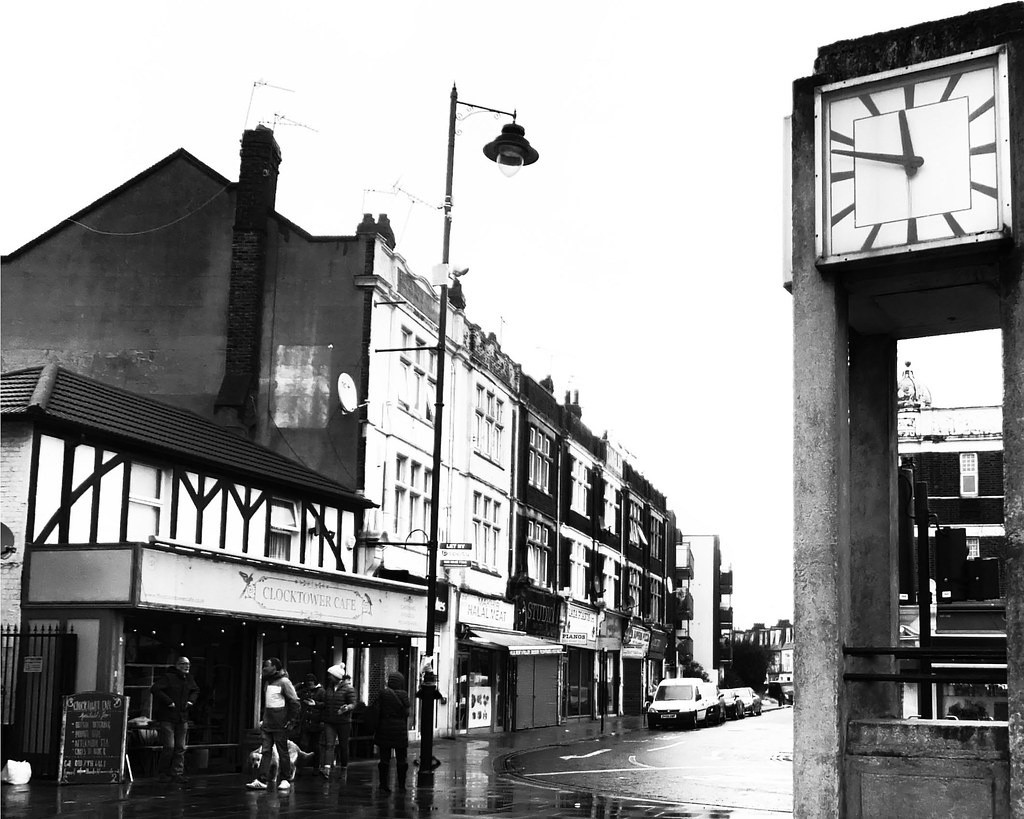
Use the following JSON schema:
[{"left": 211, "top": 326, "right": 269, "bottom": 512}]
[
  {"left": 340, "top": 704, "right": 349, "bottom": 713},
  {"left": 303, "top": 698, "right": 316, "bottom": 706}
]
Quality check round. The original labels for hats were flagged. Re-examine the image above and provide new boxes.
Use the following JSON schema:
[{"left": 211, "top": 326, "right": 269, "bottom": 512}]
[{"left": 327, "top": 662, "right": 345, "bottom": 679}]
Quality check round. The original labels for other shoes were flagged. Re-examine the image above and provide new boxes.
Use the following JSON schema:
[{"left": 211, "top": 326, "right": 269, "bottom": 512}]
[
  {"left": 171, "top": 775, "right": 188, "bottom": 783},
  {"left": 154, "top": 773, "right": 172, "bottom": 783},
  {"left": 380, "top": 787, "right": 391, "bottom": 794},
  {"left": 338, "top": 766, "right": 347, "bottom": 779},
  {"left": 320, "top": 767, "right": 330, "bottom": 779},
  {"left": 278, "top": 780, "right": 291, "bottom": 789},
  {"left": 399, "top": 787, "right": 406, "bottom": 794},
  {"left": 246, "top": 779, "right": 267, "bottom": 789},
  {"left": 312, "top": 768, "right": 319, "bottom": 775}
]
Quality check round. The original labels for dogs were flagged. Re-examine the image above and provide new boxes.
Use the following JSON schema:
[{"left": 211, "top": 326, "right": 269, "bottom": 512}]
[{"left": 246, "top": 739, "right": 314, "bottom": 781}]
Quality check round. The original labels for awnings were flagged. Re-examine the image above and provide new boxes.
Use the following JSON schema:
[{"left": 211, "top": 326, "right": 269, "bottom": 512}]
[{"left": 470, "top": 629, "right": 568, "bottom": 655}]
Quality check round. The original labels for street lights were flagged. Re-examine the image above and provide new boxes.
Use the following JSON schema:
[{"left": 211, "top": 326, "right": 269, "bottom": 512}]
[{"left": 412, "top": 84, "right": 539, "bottom": 769}]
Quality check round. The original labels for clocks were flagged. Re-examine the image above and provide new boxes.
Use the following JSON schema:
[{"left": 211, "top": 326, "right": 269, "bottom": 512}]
[{"left": 812, "top": 42, "right": 1013, "bottom": 264}]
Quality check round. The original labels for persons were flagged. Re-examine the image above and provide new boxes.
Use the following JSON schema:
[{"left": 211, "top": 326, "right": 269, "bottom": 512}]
[
  {"left": 372, "top": 672, "right": 410, "bottom": 791},
  {"left": 294, "top": 665, "right": 357, "bottom": 780},
  {"left": 245, "top": 657, "right": 301, "bottom": 790},
  {"left": 149, "top": 656, "right": 200, "bottom": 784}
]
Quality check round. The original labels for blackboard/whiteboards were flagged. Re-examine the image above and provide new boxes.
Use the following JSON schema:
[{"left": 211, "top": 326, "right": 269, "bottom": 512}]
[{"left": 58, "top": 691, "right": 130, "bottom": 775}]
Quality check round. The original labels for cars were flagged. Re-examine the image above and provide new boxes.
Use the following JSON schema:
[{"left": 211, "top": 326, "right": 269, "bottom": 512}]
[{"left": 647, "top": 677, "right": 762, "bottom": 732}]
